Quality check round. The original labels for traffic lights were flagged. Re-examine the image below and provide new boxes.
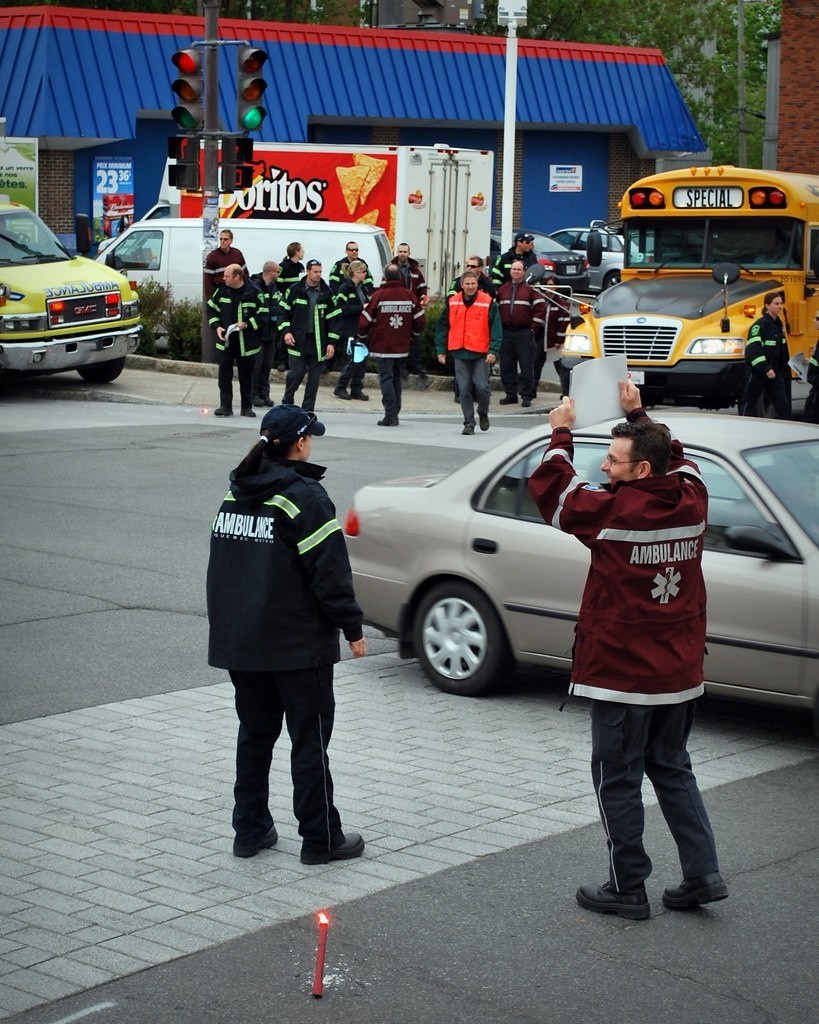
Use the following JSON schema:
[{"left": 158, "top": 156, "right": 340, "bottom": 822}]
[
  {"left": 238, "top": 46, "right": 268, "bottom": 132},
  {"left": 172, "top": 49, "right": 206, "bottom": 130},
  {"left": 166, "top": 136, "right": 202, "bottom": 191},
  {"left": 221, "top": 139, "right": 257, "bottom": 191}
]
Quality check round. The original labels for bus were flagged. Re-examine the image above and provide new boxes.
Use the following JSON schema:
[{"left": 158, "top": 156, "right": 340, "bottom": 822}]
[{"left": 524, "top": 162, "right": 818, "bottom": 417}]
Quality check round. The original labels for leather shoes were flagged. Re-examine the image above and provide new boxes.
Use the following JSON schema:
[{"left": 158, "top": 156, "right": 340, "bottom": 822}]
[
  {"left": 240, "top": 407, "right": 256, "bottom": 417},
  {"left": 214, "top": 406, "right": 233, "bottom": 416},
  {"left": 378, "top": 417, "right": 399, "bottom": 426},
  {"left": 522, "top": 400, "right": 531, "bottom": 407},
  {"left": 334, "top": 386, "right": 352, "bottom": 400},
  {"left": 663, "top": 871, "right": 729, "bottom": 908},
  {"left": 233, "top": 825, "right": 278, "bottom": 858},
  {"left": 576, "top": 880, "right": 650, "bottom": 920},
  {"left": 350, "top": 390, "right": 369, "bottom": 401},
  {"left": 500, "top": 396, "right": 518, "bottom": 405},
  {"left": 300, "top": 832, "right": 365, "bottom": 866}
]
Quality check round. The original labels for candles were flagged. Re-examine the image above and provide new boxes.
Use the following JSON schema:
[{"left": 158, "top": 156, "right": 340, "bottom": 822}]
[{"left": 310, "top": 914, "right": 331, "bottom": 999}]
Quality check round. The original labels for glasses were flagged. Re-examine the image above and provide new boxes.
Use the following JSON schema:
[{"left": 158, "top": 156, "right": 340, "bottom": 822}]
[
  {"left": 467, "top": 265, "right": 480, "bottom": 268},
  {"left": 606, "top": 454, "right": 643, "bottom": 465},
  {"left": 296, "top": 411, "right": 317, "bottom": 437},
  {"left": 220, "top": 238, "right": 228, "bottom": 241},
  {"left": 347, "top": 248, "right": 358, "bottom": 252}
]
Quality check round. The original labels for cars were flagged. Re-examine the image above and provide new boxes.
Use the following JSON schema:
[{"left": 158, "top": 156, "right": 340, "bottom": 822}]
[
  {"left": 629, "top": 232, "right": 655, "bottom": 253},
  {"left": 489, "top": 229, "right": 589, "bottom": 295},
  {"left": 343, "top": 408, "right": 818, "bottom": 719},
  {"left": 548, "top": 226, "right": 642, "bottom": 296}
]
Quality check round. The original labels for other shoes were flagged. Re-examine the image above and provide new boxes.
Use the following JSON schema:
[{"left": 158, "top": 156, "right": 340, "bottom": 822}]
[{"left": 252, "top": 398, "right": 274, "bottom": 407}]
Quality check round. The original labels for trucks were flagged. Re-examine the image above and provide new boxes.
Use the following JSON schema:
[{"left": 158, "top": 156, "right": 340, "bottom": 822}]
[
  {"left": 0, "top": 201, "right": 140, "bottom": 390},
  {"left": 96, "top": 138, "right": 493, "bottom": 301}
]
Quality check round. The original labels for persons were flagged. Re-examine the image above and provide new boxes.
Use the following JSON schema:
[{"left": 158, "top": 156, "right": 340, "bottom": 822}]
[
  {"left": 804, "top": 316, "right": 819, "bottom": 424},
  {"left": 740, "top": 292, "right": 791, "bottom": 420},
  {"left": 432, "top": 232, "right": 575, "bottom": 406},
  {"left": 528, "top": 380, "right": 730, "bottom": 919},
  {"left": 432, "top": 272, "right": 503, "bottom": 434},
  {"left": 206, "top": 403, "right": 366, "bottom": 865},
  {"left": 204, "top": 229, "right": 427, "bottom": 426}
]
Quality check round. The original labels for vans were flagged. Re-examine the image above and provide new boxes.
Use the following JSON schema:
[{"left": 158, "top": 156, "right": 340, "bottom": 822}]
[{"left": 93, "top": 219, "right": 392, "bottom": 295}]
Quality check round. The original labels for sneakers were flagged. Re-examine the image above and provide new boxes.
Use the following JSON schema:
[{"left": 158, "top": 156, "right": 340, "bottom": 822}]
[
  {"left": 479, "top": 412, "right": 489, "bottom": 431},
  {"left": 462, "top": 425, "right": 474, "bottom": 434}
]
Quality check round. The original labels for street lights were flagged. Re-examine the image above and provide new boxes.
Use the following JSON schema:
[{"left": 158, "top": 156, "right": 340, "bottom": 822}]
[{"left": 497, "top": 2, "right": 528, "bottom": 250}]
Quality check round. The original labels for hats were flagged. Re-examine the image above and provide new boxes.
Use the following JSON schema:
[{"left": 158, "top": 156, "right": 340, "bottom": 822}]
[
  {"left": 514, "top": 232, "right": 531, "bottom": 241},
  {"left": 260, "top": 404, "right": 325, "bottom": 445}
]
